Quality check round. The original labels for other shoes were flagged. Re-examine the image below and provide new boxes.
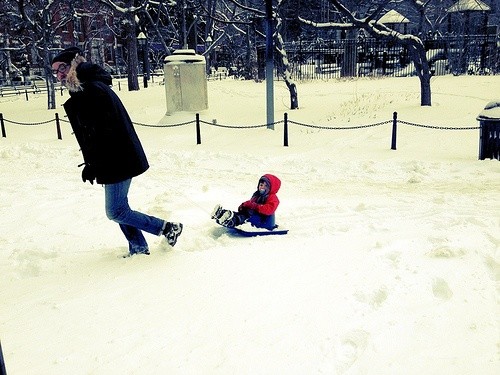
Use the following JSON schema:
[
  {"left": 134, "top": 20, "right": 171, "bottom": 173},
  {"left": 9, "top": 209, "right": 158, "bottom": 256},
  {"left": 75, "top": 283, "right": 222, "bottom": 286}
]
[
  {"left": 123, "top": 251, "right": 149, "bottom": 258},
  {"left": 166, "top": 222, "right": 183, "bottom": 246}
]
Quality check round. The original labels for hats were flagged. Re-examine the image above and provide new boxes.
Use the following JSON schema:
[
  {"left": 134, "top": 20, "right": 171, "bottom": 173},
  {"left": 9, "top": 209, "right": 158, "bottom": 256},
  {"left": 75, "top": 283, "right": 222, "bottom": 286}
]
[{"left": 52, "top": 47, "right": 84, "bottom": 67}]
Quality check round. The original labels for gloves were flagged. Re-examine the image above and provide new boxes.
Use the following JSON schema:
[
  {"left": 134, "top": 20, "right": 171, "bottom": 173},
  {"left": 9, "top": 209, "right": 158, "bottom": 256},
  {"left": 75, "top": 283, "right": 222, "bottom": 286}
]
[{"left": 82, "top": 166, "right": 97, "bottom": 185}]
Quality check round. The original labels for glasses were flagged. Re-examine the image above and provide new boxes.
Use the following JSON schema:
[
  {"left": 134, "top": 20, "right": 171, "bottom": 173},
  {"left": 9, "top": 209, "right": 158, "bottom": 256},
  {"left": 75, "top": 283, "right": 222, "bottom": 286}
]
[{"left": 51, "top": 64, "right": 69, "bottom": 78}]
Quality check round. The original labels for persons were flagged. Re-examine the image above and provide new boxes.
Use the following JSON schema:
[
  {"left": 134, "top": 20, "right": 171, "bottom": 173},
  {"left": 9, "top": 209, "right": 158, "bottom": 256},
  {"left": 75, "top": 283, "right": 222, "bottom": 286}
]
[
  {"left": 210, "top": 173, "right": 281, "bottom": 230},
  {"left": 51, "top": 46, "right": 183, "bottom": 259}
]
